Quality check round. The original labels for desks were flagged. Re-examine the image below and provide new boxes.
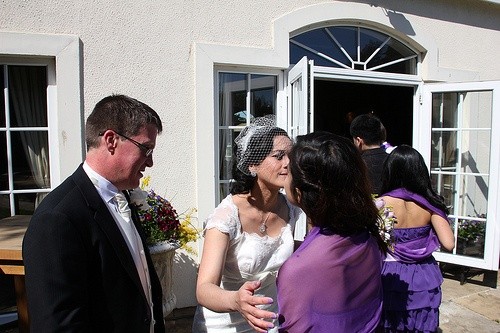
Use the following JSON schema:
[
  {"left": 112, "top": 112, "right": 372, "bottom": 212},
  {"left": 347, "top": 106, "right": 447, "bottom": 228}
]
[{"left": 0, "top": 215, "right": 33, "bottom": 332}]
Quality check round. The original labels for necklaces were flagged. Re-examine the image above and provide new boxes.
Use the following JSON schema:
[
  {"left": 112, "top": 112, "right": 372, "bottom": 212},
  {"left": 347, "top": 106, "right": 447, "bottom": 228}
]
[{"left": 248, "top": 191, "right": 280, "bottom": 233}]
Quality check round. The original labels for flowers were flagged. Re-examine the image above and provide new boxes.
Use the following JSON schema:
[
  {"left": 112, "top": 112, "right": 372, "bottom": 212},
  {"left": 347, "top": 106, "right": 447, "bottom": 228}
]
[
  {"left": 446, "top": 213, "right": 487, "bottom": 246},
  {"left": 130, "top": 176, "right": 205, "bottom": 257},
  {"left": 370, "top": 193, "right": 399, "bottom": 253}
]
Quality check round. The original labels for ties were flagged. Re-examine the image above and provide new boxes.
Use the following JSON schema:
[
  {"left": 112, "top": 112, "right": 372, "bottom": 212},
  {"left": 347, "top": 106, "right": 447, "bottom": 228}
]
[{"left": 113, "top": 192, "right": 131, "bottom": 223}]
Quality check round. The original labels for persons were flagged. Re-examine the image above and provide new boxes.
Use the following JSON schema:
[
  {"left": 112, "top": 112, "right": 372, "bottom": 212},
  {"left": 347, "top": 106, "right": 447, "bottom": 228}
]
[
  {"left": 22, "top": 94, "right": 166, "bottom": 332},
  {"left": 372, "top": 145, "right": 455, "bottom": 333},
  {"left": 193, "top": 115, "right": 312, "bottom": 333},
  {"left": 276, "top": 132, "right": 388, "bottom": 333},
  {"left": 380, "top": 121, "right": 398, "bottom": 155},
  {"left": 352, "top": 114, "right": 391, "bottom": 196}
]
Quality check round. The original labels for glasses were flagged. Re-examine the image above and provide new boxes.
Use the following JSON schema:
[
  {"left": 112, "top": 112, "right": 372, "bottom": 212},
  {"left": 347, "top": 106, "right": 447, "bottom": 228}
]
[{"left": 99, "top": 128, "right": 153, "bottom": 158}]
